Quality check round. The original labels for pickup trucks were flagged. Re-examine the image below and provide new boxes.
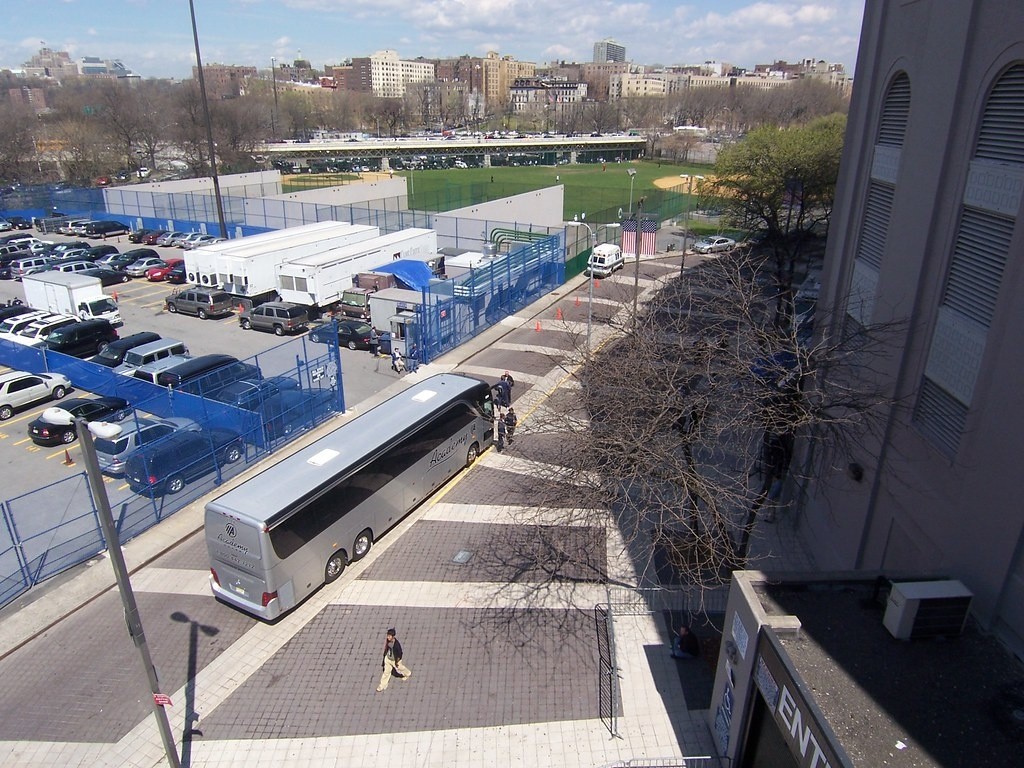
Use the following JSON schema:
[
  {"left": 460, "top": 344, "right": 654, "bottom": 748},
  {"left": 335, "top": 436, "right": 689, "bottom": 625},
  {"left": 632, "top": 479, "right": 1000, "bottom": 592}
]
[{"left": 165, "top": 286, "right": 234, "bottom": 319}]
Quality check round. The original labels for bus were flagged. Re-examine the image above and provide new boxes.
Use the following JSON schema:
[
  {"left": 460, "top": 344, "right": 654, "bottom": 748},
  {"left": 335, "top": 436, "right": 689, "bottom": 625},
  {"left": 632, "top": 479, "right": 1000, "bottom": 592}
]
[
  {"left": 673, "top": 125, "right": 706, "bottom": 140},
  {"left": 203, "top": 373, "right": 503, "bottom": 621}
]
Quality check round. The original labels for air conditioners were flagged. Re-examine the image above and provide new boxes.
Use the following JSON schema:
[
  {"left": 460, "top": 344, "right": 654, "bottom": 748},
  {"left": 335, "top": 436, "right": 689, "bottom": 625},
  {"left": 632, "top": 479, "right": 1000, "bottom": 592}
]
[{"left": 882, "top": 580, "right": 973, "bottom": 639}]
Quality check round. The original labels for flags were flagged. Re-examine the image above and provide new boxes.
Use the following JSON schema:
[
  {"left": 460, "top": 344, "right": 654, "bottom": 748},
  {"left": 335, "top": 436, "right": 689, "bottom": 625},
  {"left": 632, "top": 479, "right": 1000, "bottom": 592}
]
[{"left": 621, "top": 219, "right": 656, "bottom": 257}]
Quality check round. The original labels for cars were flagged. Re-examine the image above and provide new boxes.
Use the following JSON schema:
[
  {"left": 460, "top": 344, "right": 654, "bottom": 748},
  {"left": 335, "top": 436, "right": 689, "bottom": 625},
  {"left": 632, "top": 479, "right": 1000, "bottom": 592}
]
[
  {"left": 115, "top": 171, "right": 129, "bottom": 181},
  {"left": 0, "top": 215, "right": 228, "bottom": 286},
  {"left": 705, "top": 129, "right": 748, "bottom": 142},
  {"left": 311, "top": 320, "right": 376, "bottom": 350},
  {"left": 137, "top": 167, "right": 151, "bottom": 177},
  {"left": 255, "top": 130, "right": 641, "bottom": 174},
  {"left": 27, "top": 397, "right": 131, "bottom": 446},
  {"left": 97, "top": 176, "right": 111, "bottom": 185},
  {"left": 214, "top": 376, "right": 300, "bottom": 410},
  {"left": 253, "top": 388, "right": 334, "bottom": 442},
  {"left": 692, "top": 236, "right": 735, "bottom": 254}
]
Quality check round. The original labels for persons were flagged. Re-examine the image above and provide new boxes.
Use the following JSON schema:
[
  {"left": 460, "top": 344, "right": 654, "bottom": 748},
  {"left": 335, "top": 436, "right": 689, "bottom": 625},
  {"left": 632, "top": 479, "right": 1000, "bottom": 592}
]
[
  {"left": 392, "top": 347, "right": 405, "bottom": 374},
  {"left": 497, "top": 407, "right": 517, "bottom": 453},
  {"left": 409, "top": 343, "right": 419, "bottom": 373},
  {"left": 497, "top": 371, "right": 514, "bottom": 407},
  {"left": 376, "top": 629, "right": 412, "bottom": 693}
]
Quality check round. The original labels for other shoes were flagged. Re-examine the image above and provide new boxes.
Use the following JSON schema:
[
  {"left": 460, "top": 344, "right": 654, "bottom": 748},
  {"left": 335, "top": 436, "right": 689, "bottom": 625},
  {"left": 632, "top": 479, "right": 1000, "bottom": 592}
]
[
  {"left": 401, "top": 676, "right": 408, "bottom": 680},
  {"left": 376, "top": 687, "right": 384, "bottom": 691}
]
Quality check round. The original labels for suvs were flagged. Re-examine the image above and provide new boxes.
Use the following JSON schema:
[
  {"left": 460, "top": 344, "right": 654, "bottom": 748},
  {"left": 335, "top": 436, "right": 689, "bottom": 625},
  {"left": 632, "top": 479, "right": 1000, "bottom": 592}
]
[
  {"left": 238, "top": 303, "right": 309, "bottom": 336},
  {"left": 0, "top": 370, "right": 71, "bottom": 420},
  {"left": 93, "top": 416, "right": 204, "bottom": 476},
  {"left": 134, "top": 354, "right": 197, "bottom": 384}
]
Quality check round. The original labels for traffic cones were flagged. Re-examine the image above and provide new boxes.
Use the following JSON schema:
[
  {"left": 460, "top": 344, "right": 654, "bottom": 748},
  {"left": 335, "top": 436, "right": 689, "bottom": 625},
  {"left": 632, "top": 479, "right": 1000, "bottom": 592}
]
[
  {"left": 64, "top": 449, "right": 75, "bottom": 465},
  {"left": 535, "top": 322, "right": 541, "bottom": 332},
  {"left": 595, "top": 278, "right": 600, "bottom": 288},
  {"left": 575, "top": 296, "right": 580, "bottom": 306},
  {"left": 113, "top": 290, "right": 118, "bottom": 299},
  {"left": 239, "top": 304, "right": 243, "bottom": 314},
  {"left": 555, "top": 307, "right": 562, "bottom": 319}
]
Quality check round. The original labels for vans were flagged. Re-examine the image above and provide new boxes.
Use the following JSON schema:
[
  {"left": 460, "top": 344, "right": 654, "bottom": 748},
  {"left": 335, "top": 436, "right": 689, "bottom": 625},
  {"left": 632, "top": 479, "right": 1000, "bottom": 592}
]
[
  {"left": 31, "top": 318, "right": 120, "bottom": 358},
  {"left": 124, "top": 427, "right": 245, "bottom": 497},
  {"left": 587, "top": 243, "right": 625, "bottom": 278},
  {"left": 0, "top": 306, "right": 30, "bottom": 323},
  {"left": 15, "top": 314, "right": 82, "bottom": 346},
  {"left": 0, "top": 311, "right": 54, "bottom": 339},
  {"left": 113, "top": 337, "right": 189, "bottom": 377},
  {"left": 159, "top": 354, "right": 263, "bottom": 397},
  {"left": 88, "top": 332, "right": 161, "bottom": 368}
]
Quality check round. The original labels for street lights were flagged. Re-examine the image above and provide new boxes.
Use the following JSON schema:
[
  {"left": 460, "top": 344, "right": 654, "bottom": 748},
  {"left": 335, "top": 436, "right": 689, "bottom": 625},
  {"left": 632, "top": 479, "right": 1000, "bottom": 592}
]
[
  {"left": 626, "top": 168, "right": 636, "bottom": 213},
  {"left": 40, "top": 407, "right": 183, "bottom": 768},
  {"left": 680, "top": 174, "right": 705, "bottom": 284},
  {"left": 567, "top": 221, "right": 621, "bottom": 402}
]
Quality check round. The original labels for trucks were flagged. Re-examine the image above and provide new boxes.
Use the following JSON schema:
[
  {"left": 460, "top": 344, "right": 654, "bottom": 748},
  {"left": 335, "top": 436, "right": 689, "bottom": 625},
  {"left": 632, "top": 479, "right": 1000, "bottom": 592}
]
[
  {"left": 333, "top": 260, "right": 429, "bottom": 321},
  {"left": 21, "top": 269, "right": 120, "bottom": 327}
]
